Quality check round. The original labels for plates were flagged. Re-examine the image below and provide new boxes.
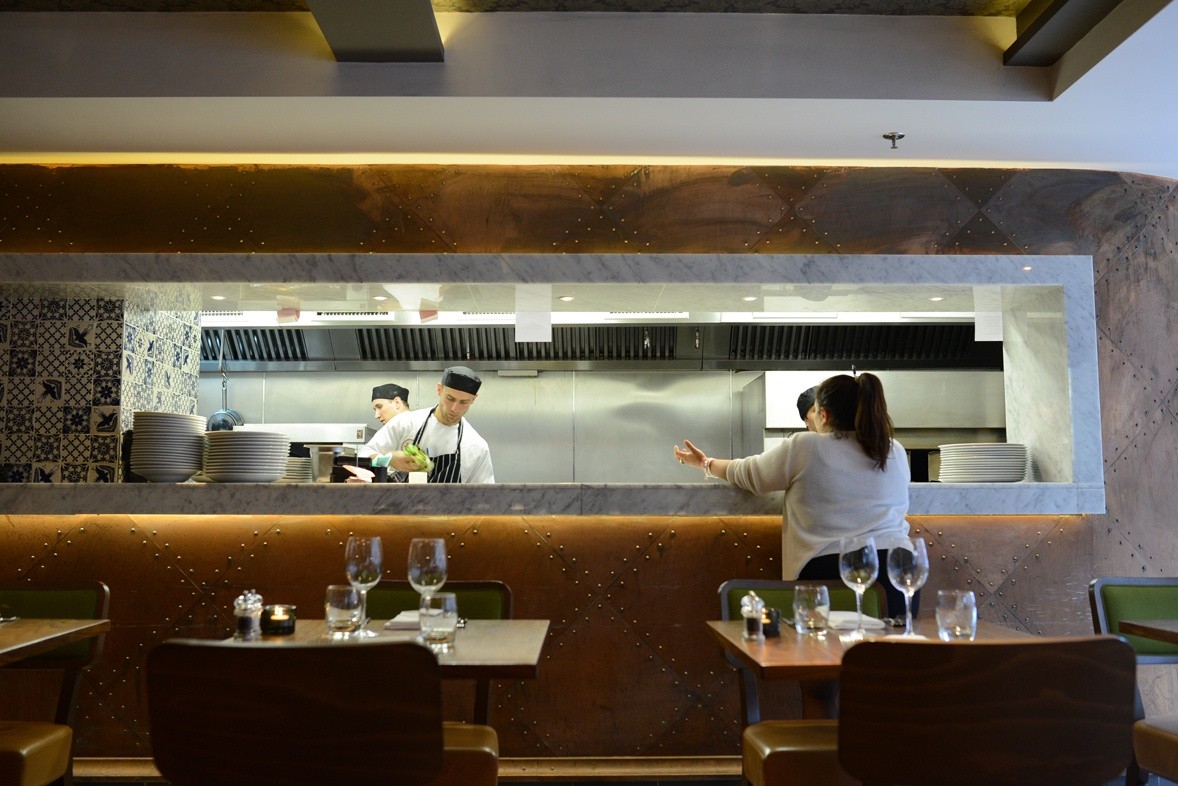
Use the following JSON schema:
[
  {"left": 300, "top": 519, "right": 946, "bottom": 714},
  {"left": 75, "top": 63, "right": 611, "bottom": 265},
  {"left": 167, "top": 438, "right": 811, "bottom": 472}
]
[
  {"left": 129, "top": 411, "right": 315, "bottom": 485},
  {"left": 938, "top": 442, "right": 1029, "bottom": 482}
]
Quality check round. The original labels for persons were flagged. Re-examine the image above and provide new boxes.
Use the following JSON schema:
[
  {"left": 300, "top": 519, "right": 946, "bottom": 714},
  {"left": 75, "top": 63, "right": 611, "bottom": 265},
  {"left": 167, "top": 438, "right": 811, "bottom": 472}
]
[
  {"left": 358, "top": 366, "right": 497, "bottom": 484},
  {"left": 674, "top": 374, "right": 921, "bottom": 719}
]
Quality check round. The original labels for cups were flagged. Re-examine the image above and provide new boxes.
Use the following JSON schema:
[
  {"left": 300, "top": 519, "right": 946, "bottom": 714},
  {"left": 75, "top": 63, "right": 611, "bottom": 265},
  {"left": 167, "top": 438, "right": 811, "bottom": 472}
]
[
  {"left": 418, "top": 592, "right": 458, "bottom": 655},
  {"left": 760, "top": 608, "right": 780, "bottom": 638},
  {"left": 325, "top": 586, "right": 367, "bottom": 640},
  {"left": 793, "top": 585, "right": 830, "bottom": 636},
  {"left": 934, "top": 589, "right": 978, "bottom": 642}
]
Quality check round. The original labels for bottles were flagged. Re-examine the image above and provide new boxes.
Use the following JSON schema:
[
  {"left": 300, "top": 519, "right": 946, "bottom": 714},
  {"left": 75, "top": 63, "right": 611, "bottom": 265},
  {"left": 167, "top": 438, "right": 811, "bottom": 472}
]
[
  {"left": 233, "top": 589, "right": 263, "bottom": 644},
  {"left": 739, "top": 594, "right": 766, "bottom": 642}
]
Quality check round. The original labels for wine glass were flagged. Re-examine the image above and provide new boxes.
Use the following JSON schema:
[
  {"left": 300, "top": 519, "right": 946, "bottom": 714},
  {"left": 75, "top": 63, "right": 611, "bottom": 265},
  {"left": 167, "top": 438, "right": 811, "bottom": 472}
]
[
  {"left": 887, "top": 536, "right": 930, "bottom": 640},
  {"left": 344, "top": 535, "right": 384, "bottom": 638},
  {"left": 406, "top": 538, "right": 448, "bottom": 608},
  {"left": 838, "top": 535, "right": 885, "bottom": 640}
]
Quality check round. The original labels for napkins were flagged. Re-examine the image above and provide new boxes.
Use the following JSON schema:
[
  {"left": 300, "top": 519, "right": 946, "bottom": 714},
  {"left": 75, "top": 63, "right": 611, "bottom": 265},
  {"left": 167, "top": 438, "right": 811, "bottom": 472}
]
[
  {"left": 828, "top": 610, "right": 886, "bottom": 630},
  {"left": 383, "top": 609, "right": 444, "bottom": 630}
]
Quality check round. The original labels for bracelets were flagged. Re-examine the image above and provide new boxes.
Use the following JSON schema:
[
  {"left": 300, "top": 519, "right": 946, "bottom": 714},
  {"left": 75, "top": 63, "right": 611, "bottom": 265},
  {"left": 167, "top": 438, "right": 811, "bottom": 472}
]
[{"left": 704, "top": 458, "right": 718, "bottom": 478}]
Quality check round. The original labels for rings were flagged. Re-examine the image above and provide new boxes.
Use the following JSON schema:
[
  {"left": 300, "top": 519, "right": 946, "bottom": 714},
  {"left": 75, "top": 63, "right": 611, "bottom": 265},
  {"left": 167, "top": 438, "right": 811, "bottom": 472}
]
[{"left": 680, "top": 458, "right": 684, "bottom": 464}]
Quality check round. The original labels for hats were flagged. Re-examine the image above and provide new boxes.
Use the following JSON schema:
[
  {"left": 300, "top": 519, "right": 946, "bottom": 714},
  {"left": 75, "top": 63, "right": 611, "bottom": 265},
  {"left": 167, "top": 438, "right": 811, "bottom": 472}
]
[
  {"left": 441, "top": 366, "right": 482, "bottom": 395},
  {"left": 796, "top": 385, "right": 819, "bottom": 420},
  {"left": 371, "top": 385, "right": 409, "bottom": 402}
]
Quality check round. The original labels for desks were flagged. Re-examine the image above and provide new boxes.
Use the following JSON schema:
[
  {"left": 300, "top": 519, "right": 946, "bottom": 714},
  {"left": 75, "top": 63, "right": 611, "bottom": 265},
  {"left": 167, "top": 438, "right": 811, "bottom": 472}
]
[
  {"left": 707, "top": 615, "right": 1026, "bottom": 681},
  {"left": 223, "top": 619, "right": 552, "bottom": 679},
  {"left": 0, "top": 616, "right": 111, "bottom": 668}
]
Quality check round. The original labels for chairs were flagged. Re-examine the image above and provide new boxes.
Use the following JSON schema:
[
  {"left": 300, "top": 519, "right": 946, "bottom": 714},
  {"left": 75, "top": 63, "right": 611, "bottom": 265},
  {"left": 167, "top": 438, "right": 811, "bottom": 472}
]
[
  {"left": 1088, "top": 576, "right": 1178, "bottom": 786},
  {"left": 837, "top": 634, "right": 1137, "bottom": 786},
  {"left": 143, "top": 635, "right": 445, "bottom": 786},
  {"left": 0, "top": 578, "right": 110, "bottom": 786},
  {"left": 347, "top": 581, "right": 513, "bottom": 786},
  {"left": 718, "top": 580, "right": 887, "bottom": 785}
]
